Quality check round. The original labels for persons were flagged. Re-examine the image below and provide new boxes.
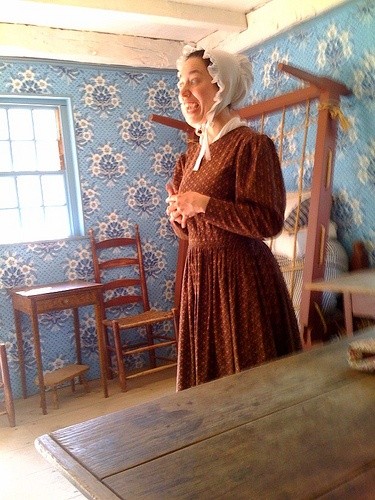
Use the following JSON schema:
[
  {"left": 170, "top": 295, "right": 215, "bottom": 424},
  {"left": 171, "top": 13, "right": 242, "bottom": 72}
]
[{"left": 163, "top": 44, "right": 304, "bottom": 395}]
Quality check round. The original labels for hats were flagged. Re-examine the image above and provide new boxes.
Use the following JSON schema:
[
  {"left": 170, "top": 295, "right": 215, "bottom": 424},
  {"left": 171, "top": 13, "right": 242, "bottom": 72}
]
[{"left": 176, "top": 44, "right": 254, "bottom": 172}]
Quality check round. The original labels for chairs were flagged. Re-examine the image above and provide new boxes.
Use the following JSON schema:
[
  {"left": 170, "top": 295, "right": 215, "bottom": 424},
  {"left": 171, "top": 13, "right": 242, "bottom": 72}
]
[
  {"left": 260, "top": 191, "right": 348, "bottom": 329},
  {"left": 0, "top": 344, "right": 17, "bottom": 427},
  {"left": 90, "top": 224, "right": 180, "bottom": 393}
]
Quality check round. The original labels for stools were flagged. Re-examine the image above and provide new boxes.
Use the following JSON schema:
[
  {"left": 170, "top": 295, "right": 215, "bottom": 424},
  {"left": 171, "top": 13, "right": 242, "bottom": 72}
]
[{"left": 35, "top": 363, "right": 91, "bottom": 409}]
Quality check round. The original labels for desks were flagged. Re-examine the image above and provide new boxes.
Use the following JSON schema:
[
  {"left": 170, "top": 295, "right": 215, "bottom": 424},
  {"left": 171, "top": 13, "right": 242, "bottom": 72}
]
[
  {"left": 304, "top": 267, "right": 374, "bottom": 340},
  {"left": 33, "top": 326, "right": 375, "bottom": 499},
  {"left": 7, "top": 279, "right": 109, "bottom": 416}
]
